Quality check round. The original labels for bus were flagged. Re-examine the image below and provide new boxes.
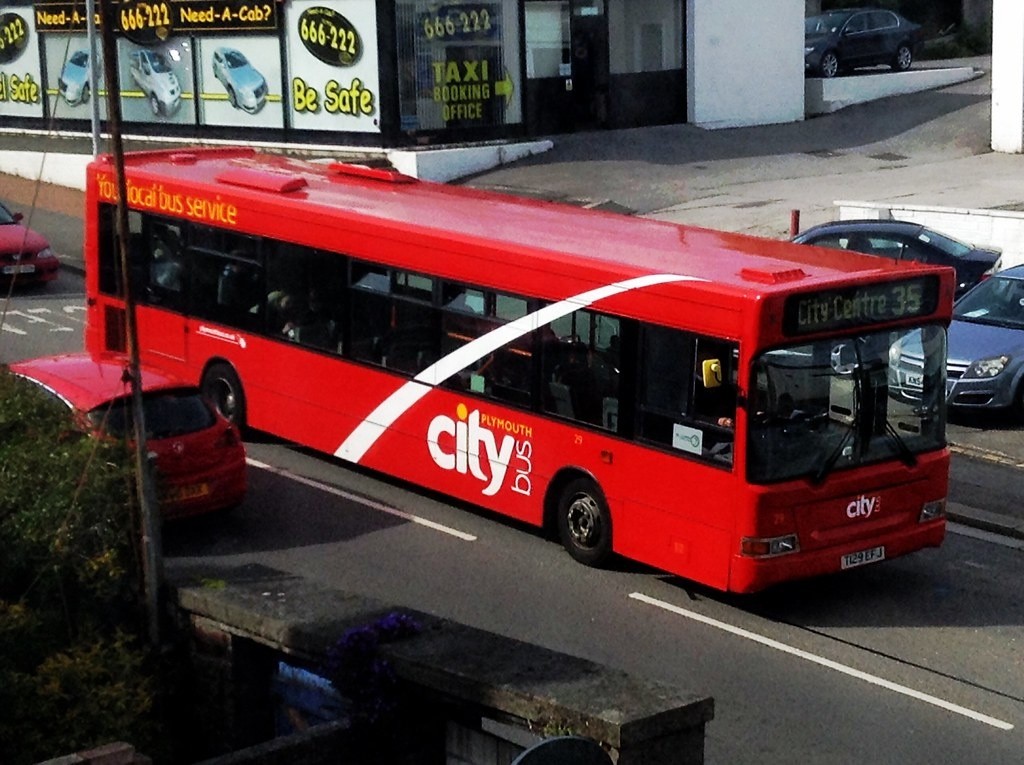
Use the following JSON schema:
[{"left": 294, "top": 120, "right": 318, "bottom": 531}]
[{"left": 85, "top": 145, "right": 955, "bottom": 594}]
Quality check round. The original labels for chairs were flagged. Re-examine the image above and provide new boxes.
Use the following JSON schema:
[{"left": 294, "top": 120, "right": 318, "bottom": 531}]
[
  {"left": 336, "top": 325, "right": 380, "bottom": 359},
  {"left": 382, "top": 341, "right": 424, "bottom": 371}
]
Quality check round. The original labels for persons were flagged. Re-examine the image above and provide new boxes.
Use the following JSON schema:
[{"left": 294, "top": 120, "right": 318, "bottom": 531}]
[
  {"left": 153, "top": 229, "right": 302, "bottom": 342},
  {"left": 690, "top": 348, "right": 735, "bottom": 451},
  {"left": 776, "top": 393, "right": 805, "bottom": 420}
]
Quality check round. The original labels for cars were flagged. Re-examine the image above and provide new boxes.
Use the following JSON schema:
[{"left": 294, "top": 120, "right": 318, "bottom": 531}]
[
  {"left": 788, "top": 219, "right": 1002, "bottom": 374},
  {"left": 58, "top": 50, "right": 101, "bottom": 107},
  {"left": 804, "top": 7, "right": 925, "bottom": 78},
  {"left": 213, "top": 48, "right": 269, "bottom": 115},
  {"left": 130, "top": 49, "right": 184, "bottom": 117},
  {"left": 0, "top": 203, "right": 61, "bottom": 291},
  {"left": 887, "top": 264, "right": 1024, "bottom": 408},
  {"left": 4, "top": 352, "right": 250, "bottom": 521}
]
